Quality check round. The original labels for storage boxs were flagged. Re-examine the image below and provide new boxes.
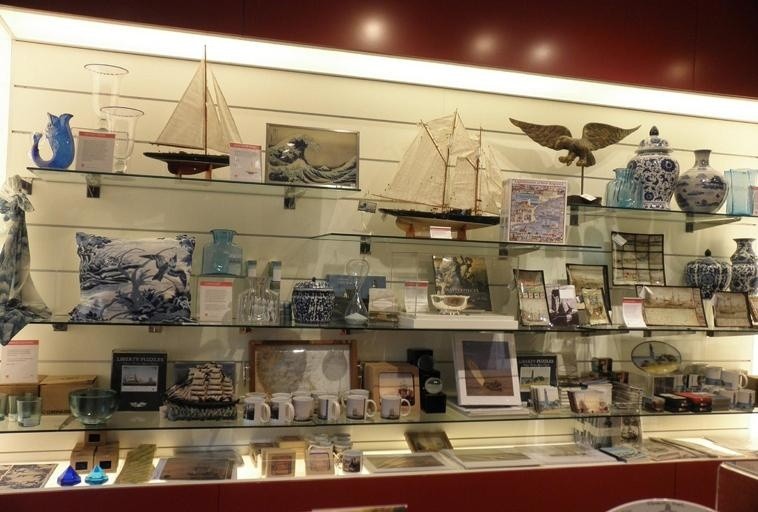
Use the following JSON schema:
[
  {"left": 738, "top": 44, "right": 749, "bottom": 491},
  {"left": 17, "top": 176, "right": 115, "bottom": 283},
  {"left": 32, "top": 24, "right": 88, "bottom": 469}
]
[
  {"left": 40, "top": 376, "right": 98, "bottom": 411},
  {"left": 0, "top": 374, "right": 46, "bottom": 412}
]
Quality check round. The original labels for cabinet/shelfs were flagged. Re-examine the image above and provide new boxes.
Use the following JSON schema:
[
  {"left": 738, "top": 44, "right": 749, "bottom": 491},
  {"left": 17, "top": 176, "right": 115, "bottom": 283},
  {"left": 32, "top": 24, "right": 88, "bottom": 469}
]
[{"left": 0, "top": 167, "right": 758, "bottom": 434}]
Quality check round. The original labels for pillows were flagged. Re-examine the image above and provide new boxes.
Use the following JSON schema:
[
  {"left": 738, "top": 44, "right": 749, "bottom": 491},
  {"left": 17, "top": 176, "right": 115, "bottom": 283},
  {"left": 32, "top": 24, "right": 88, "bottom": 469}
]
[{"left": 71, "top": 230, "right": 197, "bottom": 326}]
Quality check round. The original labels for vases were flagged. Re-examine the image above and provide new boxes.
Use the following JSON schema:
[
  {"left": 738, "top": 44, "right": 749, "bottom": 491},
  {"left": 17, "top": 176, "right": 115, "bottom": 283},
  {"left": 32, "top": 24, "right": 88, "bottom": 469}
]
[
  {"left": 729, "top": 238, "right": 758, "bottom": 296},
  {"left": 673, "top": 149, "right": 729, "bottom": 216}
]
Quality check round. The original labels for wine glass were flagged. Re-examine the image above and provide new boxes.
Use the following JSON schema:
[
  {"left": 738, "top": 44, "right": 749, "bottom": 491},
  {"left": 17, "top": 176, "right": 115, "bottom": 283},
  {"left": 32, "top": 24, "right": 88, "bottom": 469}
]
[{"left": 83, "top": 62, "right": 143, "bottom": 175}]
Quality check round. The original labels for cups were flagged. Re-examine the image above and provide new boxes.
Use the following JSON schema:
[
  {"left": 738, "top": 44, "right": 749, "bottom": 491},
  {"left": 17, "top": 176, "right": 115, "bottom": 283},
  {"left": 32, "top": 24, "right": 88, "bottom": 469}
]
[
  {"left": 306, "top": 432, "right": 364, "bottom": 473},
  {"left": 241, "top": 389, "right": 411, "bottom": 425},
  {"left": 704, "top": 365, "right": 756, "bottom": 410},
  {"left": 0, "top": 391, "right": 44, "bottom": 428}
]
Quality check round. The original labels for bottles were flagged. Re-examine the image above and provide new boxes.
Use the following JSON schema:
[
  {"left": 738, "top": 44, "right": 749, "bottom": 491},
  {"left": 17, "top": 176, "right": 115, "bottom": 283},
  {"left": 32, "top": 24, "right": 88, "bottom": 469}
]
[
  {"left": 604, "top": 168, "right": 644, "bottom": 208},
  {"left": 239, "top": 276, "right": 277, "bottom": 324}
]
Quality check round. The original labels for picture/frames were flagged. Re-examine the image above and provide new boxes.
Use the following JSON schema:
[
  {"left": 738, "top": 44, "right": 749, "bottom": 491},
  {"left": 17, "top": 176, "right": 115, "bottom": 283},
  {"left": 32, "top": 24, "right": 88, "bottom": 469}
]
[
  {"left": 245, "top": 336, "right": 360, "bottom": 394},
  {"left": 111, "top": 347, "right": 168, "bottom": 413},
  {"left": 361, "top": 451, "right": 459, "bottom": 476},
  {"left": 565, "top": 263, "right": 610, "bottom": 311},
  {"left": 445, "top": 447, "right": 540, "bottom": 470},
  {"left": 404, "top": 430, "right": 455, "bottom": 453},
  {"left": 264, "top": 122, "right": 360, "bottom": 189},
  {"left": 363, "top": 358, "right": 423, "bottom": 419},
  {"left": 448, "top": 333, "right": 524, "bottom": 409},
  {"left": 251, "top": 435, "right": 336, "bottom": 481},
  {"left": 172, "top": 358, "right": 238, "bottom": 393},
  {"left": 516, "top": 348, "right": 615, "bottom": 415}
]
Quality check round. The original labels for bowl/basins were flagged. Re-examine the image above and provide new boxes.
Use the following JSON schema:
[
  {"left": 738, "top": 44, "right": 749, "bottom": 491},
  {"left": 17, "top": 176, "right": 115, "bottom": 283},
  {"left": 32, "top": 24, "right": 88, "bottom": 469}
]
[{"left": 67, "top": 389, "right": 119, "bottom": 425}]
